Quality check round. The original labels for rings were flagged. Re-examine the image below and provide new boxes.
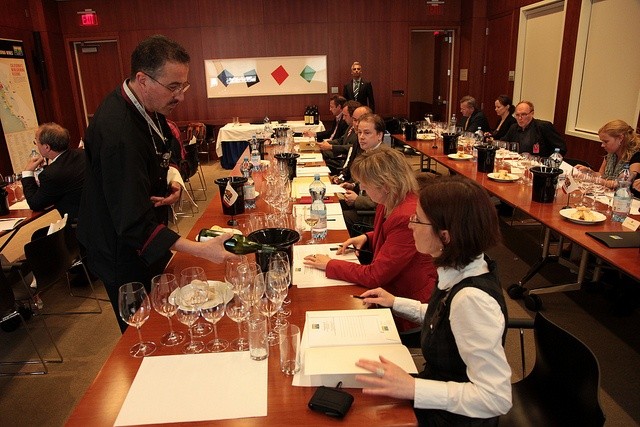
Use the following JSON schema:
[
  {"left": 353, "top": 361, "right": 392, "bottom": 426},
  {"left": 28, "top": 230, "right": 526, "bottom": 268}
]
[
  {"left": 313, "top": 255, "right": 316, "bottom": 258},
  {"left": 376, "top": 367, "right": 385, "bottom": 376}
]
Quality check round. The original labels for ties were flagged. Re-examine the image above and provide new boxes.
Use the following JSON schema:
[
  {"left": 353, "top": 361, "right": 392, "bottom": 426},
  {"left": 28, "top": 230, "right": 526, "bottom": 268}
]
[{"left": 354, "top": 81, "right": 359, "bottom": 100}]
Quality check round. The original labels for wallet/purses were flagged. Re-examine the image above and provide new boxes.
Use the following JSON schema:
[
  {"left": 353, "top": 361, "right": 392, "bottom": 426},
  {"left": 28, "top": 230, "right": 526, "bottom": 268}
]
[{"left": 309, "top": 382, "right": 354, "bottom": 420}]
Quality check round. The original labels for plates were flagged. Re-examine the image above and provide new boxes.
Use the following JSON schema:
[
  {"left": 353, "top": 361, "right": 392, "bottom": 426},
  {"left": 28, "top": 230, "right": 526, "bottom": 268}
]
[
  {"left": 416, "top": 133, "right": 439, "bottom": 141},
  {"left": 558, "top": 207, "right": 608, "bottom": 226},
  {"left": 244, "top": 189, "right": 259, "bottom": 200},
  {"left": 447, "top": 153, "right": 474, "bottom": 161},
  {"left": 167, "top": 280, "right": 234, "bottom": 311},
  {"left": 487, "top": 172, "right": 520, "bottom": 183}
]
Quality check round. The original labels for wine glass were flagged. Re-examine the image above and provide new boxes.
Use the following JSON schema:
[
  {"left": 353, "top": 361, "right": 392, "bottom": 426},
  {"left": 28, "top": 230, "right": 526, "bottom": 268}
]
[
  {"left": 401, "top": 122, "right": 408, "bottom": 137},
  {"left": 233, "top": 116, "right": 239, "bottom": 127},
  {"left": 269, "top": 260, "right": 291, "bottom": 318},
  {"left": 604, "top": 176, "right": 620, "bottom": 215},
  {"left": 256, "top": 274, "right": 282, "bottom": 347},
  {"left": 200, "top": 286, "right": 224, "bottom": 352},
  {"left": 151, "top": 274, "right": 185, "bottom": 346},
  {"left": 573, "top": 166, "right": 593, "bottom": 209},
  {"left": 180, "top": 266, "right": 213, "bottom": 337},
  {"left": 118, "top": 282, "right": 157, "bottom": 358},
  {"left": 593, "top": 174, "right": 606, "bottom": 211},
  {"left": 308, "top": 138, "right": 318, "bottom": 157},
  {"left": 268, "top": 252, "right": 290, "bottom": 286},
  {"left": 418, "top": 121, "right": 446, "bottom": 130},
  {"left": 4, "top": 174, "right": 20, "bottom": 204},
  {"left": 175, "top": 291, "right": 205, "bottom": 353},
  {"left": 557, "top": 172, "right": 577, "bottom": 212},
  {"left": 309, "top": 173, "right": 326, "bottom": 238},
  {"left": 238, "top": 214, "right": 293, "bottom": 235},
  {"left": 492, "top": 140, "right": 519, "bottom": 153},
  {"left": 304, "top": 206, "right": 319, "bottom": 245},
  {"left": 575, "top": 171, "right": 592, "bottom": 207},
  {"left": 236, "top": 262, "right": 264, "bottom": 331},
  {"left": 14, "top": 171, "right": 26, "bottom": 199},
  {"left": 226, "top": 285, "right": 251, "bottom": 351},
  {"left": 273, "top": 133, "right": 294, "bottom": 154},
  {"left": 262, "top": 162, "right": 290, "bottom": 213},
  {"left": 458, "top": 127, "right": 474, "bottom": 138},
  {"left": 521, "top": 151, "right": 549, "bottom": 164},
  {"left": 224, "top": 254, "right": 251, "bottom": 293},
  {"left": 266, "top": 270, "right": 288, "bottom": 331}
]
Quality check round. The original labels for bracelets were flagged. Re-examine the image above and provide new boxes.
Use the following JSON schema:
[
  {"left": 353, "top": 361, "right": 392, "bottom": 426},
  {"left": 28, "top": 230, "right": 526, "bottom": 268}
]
[{"left": 364, "top": 233, "right": 370, "bottom": 241}]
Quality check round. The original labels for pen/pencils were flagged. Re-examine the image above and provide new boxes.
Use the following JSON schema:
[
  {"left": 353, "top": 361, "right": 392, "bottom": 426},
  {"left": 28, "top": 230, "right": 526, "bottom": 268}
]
[
  {"left": 330, "top": 248, "right": 341, "bottom": 250},
  {"left": 327, "top": 218, "right": 337, "bottom": 221},
  {"left": 351, "top": 295, "right": 365, "bottom": 299},
  {"left": 301, "top": 157, "right": 317, "bottom": 159},
  {"left": 339, "top": 245, "right": 368, "bottom": 252}
]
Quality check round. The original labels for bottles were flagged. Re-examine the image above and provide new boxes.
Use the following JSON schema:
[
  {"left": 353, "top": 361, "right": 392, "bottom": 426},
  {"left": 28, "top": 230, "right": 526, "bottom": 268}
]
[
  {"left": 612, "top": 163, "right": 631, "bottom": 222},
  {"left": 252, "top": 143, "right": 258, "bottom": 157},
  {"left": 240, "top": 157, "right": 253, "bottom": 178},
  {"left": 475, "top": 127, "right": 484, "bottom": 146},
  {"left": 29, "top": 148, "right": 42, "bottom": 172},
  {"left": 449, "top": 114, "right": 457, "bottom": 137},
  {"left": 245, "top": 176, "right": 256, "bottom": 208},
  {"left": 264, "top": 115, "right": 272, "bottom": 139},
  {"left": 250, "top": 135, "right": 258, "bottom": 150},
  {"left": 304, "top": 104, "right": 319, "bottom": 125},
  {"left": 548, "top": 148, "right": 564, "bottom": 169},
  {"left": 198, "top": 229, "right": 278, "bottom": 254}
]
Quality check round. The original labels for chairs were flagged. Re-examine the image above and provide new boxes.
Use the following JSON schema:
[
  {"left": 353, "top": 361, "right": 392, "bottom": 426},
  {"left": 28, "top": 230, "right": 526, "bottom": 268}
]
[
  {"left": 182, "top": 136, "right": 207, "bottom": 202},
  {"left": 14, "top": 214, "right": 103, "bottom": 363},
  {"left": 174, "top": 141, "right": 208, "bottom": 220},
  {"left": 494, "top": 309, "right": 606, "bottom": 426},
  {"left": 167, "top": 166, "right": 199, "bottom": 225},
  {"left": 0, "top": 260, "right": 48, "bottom": 382}
]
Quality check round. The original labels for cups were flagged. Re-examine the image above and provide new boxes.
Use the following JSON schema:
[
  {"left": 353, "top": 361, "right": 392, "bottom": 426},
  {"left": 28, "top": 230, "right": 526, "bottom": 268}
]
[
  {"left": 279, "top": 324, "right": 300, "bottom": 376},
  {"left": 248, "top": 314, "right": 269, "bottom": 360}
]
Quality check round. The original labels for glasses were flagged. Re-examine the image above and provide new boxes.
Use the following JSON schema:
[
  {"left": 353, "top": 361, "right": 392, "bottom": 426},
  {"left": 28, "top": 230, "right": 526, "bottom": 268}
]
[
  {"left": 410, "top": 214, "right": 432, "bottom": 226},
  {"left": 141, "top": 70, "right": 192, "bottom": 97},
  {"left": 514, "top": 111, "right": 533, "bottom": 117}
]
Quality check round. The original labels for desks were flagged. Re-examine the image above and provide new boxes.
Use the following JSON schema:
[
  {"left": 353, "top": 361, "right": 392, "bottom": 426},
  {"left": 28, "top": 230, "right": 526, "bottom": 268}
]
[{"left": 214, "top": 119, "right": 326, "bottom": 170}]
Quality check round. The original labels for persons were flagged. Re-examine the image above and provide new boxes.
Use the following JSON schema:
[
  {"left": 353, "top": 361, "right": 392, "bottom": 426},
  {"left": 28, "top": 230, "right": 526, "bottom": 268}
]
[
  {"left": 354, "top": 174, "right": 513, "bottom": 426},
  {"left": 339, "top": 113, "right": 386, "bottom": 234},
  {"left": 91, "top": 34, "right": 239, "bottom": 335},
  {"left": 456, "top": 96, "right": 489, "bottom": 135},
  {"left": 500, "top": 100, "right": 568, "bottom": 163},
  {"left": 303, "top": 147, "right": 438, "bottom": 304},
  {"left": 574, "top": 120, "right": 640, "bottom": 190},
  {"left": 303, "top": 95, "right": 347, "bottom": 142},
  {"left": 164, "top": 117, "right": 186, "bottom": 161},
  {"left": 343, "top": 61, "right": 375, "bottom": 115},
  {"left": 21, "top": 122, "right": 90, "bottom": 289},
  {"left": 478, "top": 96, "right": 517, "bottom": 143},
  {"left": 629, "top": 149, "right": 640, "bottom": 199},
  {"left": 328, "top": 105, "right": 373, "bottom": 184},
  {"left": 316, "top": 100, "right": 361, "bottom": 167}
]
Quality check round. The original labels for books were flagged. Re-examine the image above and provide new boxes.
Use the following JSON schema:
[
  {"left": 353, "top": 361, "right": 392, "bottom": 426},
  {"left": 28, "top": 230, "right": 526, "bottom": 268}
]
[{"left": 585, "top": 232, "right": 640, "bottom": 248}]
